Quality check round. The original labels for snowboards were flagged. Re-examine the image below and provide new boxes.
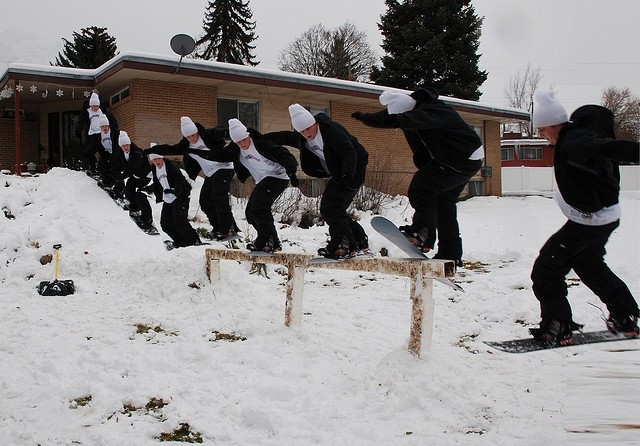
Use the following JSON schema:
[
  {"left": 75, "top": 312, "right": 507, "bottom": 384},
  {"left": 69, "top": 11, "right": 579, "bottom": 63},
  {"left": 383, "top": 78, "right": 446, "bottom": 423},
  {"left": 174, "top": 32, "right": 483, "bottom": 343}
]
[
  {"left": 84, "top": 169, "right": 129, "bottom": 210},
  {"left": 483, "top": 328, "right": 640, "bottom": 353},
  {"left": 164, "top": 240, "right": 210, "bottom": 251},
  {"left": 218, "top": 233, "right": 244, "bottom": 243},
  {"left": 370, "top": 216, "right": 465, "bottom": 294},
  {"left": 127, "top": 210, "right": 160, "bottom": 235},
  {"left": 306, "top": 247, "right": 368, "bottom": 264},
  {"left": 245, "top": 243, "right": 276, "bottom": 258}
]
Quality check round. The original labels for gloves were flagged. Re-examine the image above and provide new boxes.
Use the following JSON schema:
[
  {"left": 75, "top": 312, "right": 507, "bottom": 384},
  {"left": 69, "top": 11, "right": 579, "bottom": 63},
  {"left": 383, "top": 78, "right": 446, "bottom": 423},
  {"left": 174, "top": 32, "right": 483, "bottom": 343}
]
[
  {"left": 142, "top": 147, "right": 150, "bottom": 155},
  {"left": 138, "top": 185, "right": 146, "bottom": 191},
  {"left": 290, "top": 173, "right": 299, "bottom": 186},
  {"left": 185, "top": 147, "right": 198, "bottom": 155},
  {"left": 351, "top": 110, "right": 364, "bottom": 120},
  {"left": 75, "top": 129, "right": 82, "bottom": 137},
  {"left": 164, "top": 188, "right": 174, "bottom": 194},
  {"left": 118, "top": 172, "right": 125, "bottom": 179}
]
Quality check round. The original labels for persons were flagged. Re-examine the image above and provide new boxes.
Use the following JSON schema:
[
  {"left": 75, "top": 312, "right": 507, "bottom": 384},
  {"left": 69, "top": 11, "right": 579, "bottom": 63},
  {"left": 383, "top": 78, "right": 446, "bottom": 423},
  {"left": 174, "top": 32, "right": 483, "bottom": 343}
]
[
  {"left": 350, "top": 84, "right": 485, "bottom": 268},
  {"left": 137, "top": 141, "right": 201, "bottom": 249},
  {"left": 251, "top": 102, "right": 370, "bottom": 259},
  {"left": 180, "top": 117, "right": 299, "bottom": 255},
  {"left": 528, "top": 84, "right": 640, "bottom": 346},
  {"left": 141, "top": 115, "right": 244, "bottom": 241},
  {"left": 76, "top": 92, "right": 117, "bottom": 177},
  {"left": 95, "top": 114, "right": 118, "bottom": 193},
  {"left": 118, "top": 130, "right": 155, "bottom": 230}
]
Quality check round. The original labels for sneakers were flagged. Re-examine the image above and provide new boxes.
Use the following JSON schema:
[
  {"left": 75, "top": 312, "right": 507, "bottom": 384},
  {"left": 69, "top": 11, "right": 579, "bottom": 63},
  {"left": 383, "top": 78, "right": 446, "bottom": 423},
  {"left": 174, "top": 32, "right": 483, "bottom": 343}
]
[
  {"left": 432, "top": 254, "right": 463, "bottom": 266},
  {"left": 263, "top": 235, "right": 282, "bottom": 252},
  {"left": 315, "top": 242, "right": 337, "bottom": 257},
  {"left": 215, "top": 225, "right": 236, "bottom": 240},
  {"left": 144, "top": 222, "right": 159, "bottom": 233},
  {"left": 194, "top": 235, "right": 201, "bottom": 245},
  {"left": 246, "top": 236, "right": 268, "bottom": 251},
  {"left": 334, "top": 236, "right": 371, "bottom": 257},
  {"left": 398, "top": 224, "right": 430, "bottom": 253},
  {"left": 529, "top": 317, "right": 584, "bottom": 345},
  {"left": 207, "top": 227, "right": 220, "bottom": 239},
  {"left": 166, "top": 241, "right": 180, "bottom": 249},
  {"left": 605, "top": 311, "right": 640, "bottom": 339}
]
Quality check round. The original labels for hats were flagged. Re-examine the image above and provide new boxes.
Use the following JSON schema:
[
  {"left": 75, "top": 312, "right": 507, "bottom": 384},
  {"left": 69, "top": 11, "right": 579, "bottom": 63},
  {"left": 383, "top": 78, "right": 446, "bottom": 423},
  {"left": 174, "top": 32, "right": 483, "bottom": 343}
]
[
  {"left": 288, "top": 103, "right": 316, "bottom": 132},
  {"left": 98, "top": 113, "right": 110, "bottom": 126},
  {"left": 118, "top": 130, "right": 131, "bottom": 146},
  {"left": 378, "top": 88, "right": 416, "bottom": 113},
  {"left": 89, "top": 92, "right": 100, "bottom": 106},
  {"left": 181, "top": 115, "right": 199, "bottom": 137},
  {"left": 530, "top": 86, "right": 569, "bottom": 127},
  {"left": 149, "top": 142, "right": 163, "bottom": 160},
  {"left": 228, "top": 117, "right": 250, "bottom": 143}
]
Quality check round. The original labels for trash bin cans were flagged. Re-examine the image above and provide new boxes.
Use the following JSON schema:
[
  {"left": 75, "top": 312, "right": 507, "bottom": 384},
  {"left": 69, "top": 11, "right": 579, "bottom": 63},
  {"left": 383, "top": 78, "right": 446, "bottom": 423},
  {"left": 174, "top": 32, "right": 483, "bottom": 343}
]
[{"left": 468, "top": 176, "right": 484, "bottom": 196}]
